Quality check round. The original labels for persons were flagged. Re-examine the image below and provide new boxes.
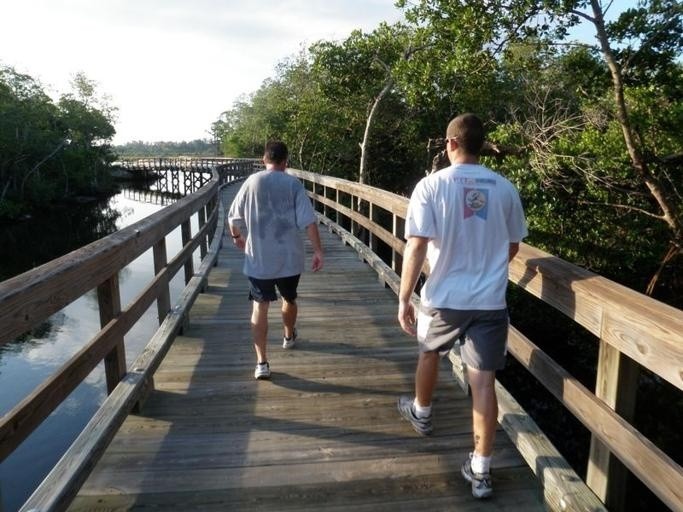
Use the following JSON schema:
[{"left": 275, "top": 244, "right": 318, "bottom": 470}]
[
  {"left": 225, "top": 139, "right": 324, "bottom": 380},
  {"left": 397, "top": 112, "right": 529, "bottom": 502}
]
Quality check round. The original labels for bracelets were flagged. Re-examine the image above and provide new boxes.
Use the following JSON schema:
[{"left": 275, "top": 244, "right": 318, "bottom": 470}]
[{"left": 231, "top": 233, "right": 241, "bottom": 239}]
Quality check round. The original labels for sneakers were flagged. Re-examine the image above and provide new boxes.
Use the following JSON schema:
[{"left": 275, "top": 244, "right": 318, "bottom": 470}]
[
  {"left": 283, "top": 328, "right": 297, "bottom": 349},
  {"left": 254, "top": 361, "right": 271, "bottom": 379},
  {"left": 397, "top": 394, "right": 434, "bottom": 435},
  {"left": 460, "top": 452, "right": 493, "bottom": 498}
]
